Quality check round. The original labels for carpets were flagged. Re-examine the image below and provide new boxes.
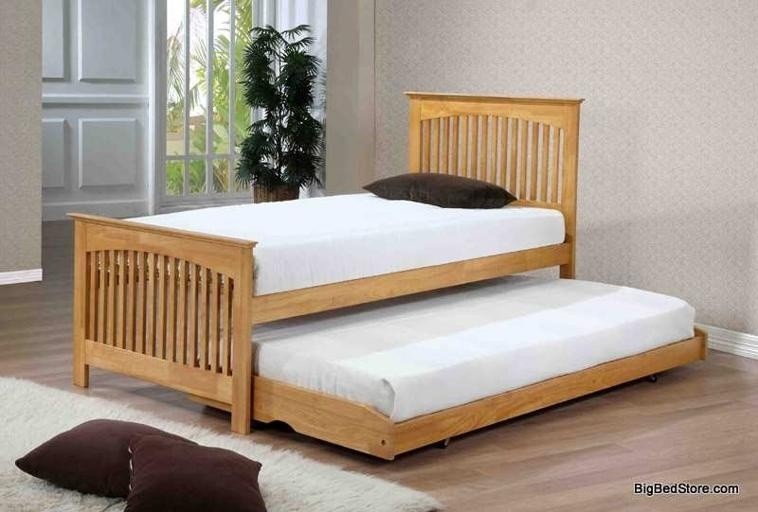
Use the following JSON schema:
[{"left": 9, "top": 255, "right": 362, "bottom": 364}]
[{"left": 0, "top": 377, "right": 444, "bottom": 512}]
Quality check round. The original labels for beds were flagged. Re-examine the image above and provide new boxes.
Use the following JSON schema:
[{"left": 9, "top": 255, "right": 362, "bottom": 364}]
[{"left": 66, "top": 90, "right": 707, "bottom": 465}]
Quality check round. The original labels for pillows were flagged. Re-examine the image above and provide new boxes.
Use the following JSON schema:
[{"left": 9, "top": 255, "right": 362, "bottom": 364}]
[
  {"left": 362, "top": 172, "right": 517, "bottom": 210},
  {"left": 14, "top": 418, "right": 266, "bottom": 512}
]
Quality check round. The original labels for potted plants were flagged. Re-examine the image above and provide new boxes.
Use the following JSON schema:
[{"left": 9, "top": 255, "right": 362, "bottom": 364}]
[{"left": 236, "top": 23, "right": 327, "bottom": 203}]
